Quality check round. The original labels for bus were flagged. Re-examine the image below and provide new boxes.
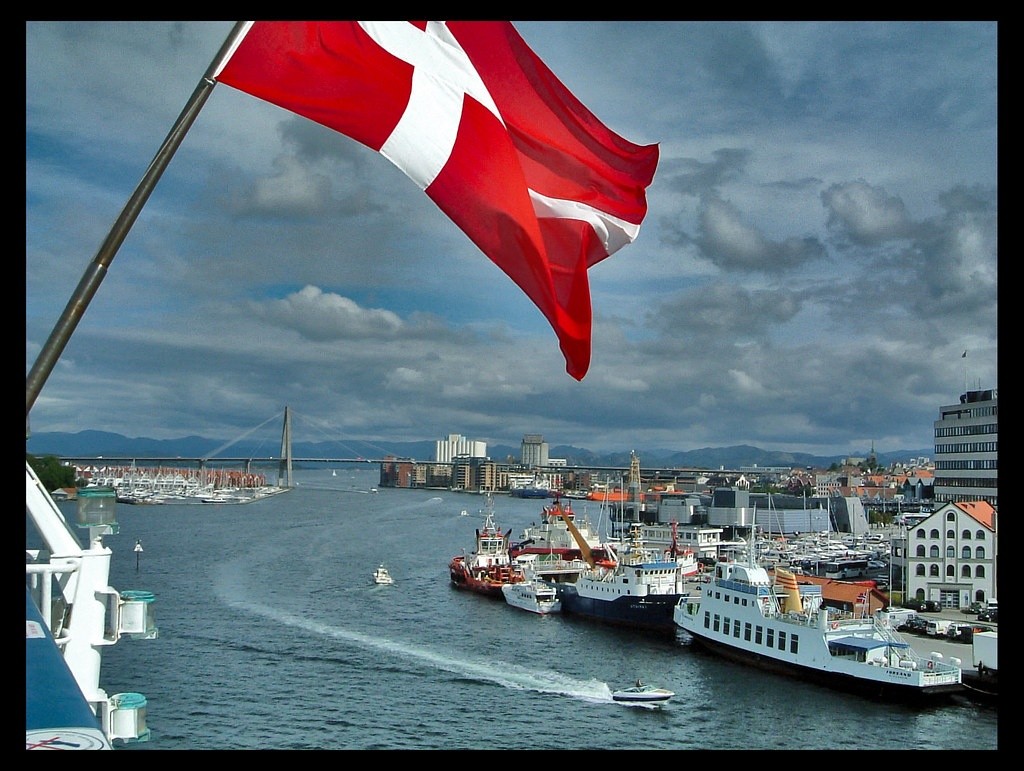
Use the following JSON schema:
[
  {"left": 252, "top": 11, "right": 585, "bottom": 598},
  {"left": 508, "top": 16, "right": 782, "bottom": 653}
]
[{"left": 824, "top": 559, "right": 871, "bottom": 580}]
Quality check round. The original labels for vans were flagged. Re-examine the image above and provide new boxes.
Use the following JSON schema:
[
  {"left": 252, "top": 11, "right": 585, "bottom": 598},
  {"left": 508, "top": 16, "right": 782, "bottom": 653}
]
[
  {"left": 926, "top": 620, "right": 957, "bottom": 639},
  {"left": 948, "top": 623, "right": 973, "bottom": 641},
  {"left": 961, "top": 625, "right": 994, "bottom": 644}
]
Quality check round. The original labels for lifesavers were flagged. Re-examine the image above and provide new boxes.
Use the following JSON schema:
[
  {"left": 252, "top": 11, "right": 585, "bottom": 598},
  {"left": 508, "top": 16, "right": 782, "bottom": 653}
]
[
  {"left": 927, "top": 661, "right": 934, "bottom": 670},
  {"left": 831, "top": 622, "right": 838, "bottom": 630}
]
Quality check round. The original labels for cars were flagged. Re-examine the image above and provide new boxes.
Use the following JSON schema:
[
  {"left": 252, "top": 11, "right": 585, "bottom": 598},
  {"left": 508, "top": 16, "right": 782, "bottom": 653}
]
[{"left": 978, "top": 611, "right": 998, "bottom": 623}]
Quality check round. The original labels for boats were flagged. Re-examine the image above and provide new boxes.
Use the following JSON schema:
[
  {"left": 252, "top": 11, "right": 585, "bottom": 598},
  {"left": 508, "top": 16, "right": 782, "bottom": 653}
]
[
  {"left": 115, "top": 484, "right": 291, "bottom": 505},
  {"left": 613, "top": 678, "right": 673, "bottom": 708},
  {"left": 443, "top": 452, "right": 964, "bottom": 704},
  {"left": 372, "top": 564, "right": 396, "bottom": 585}
]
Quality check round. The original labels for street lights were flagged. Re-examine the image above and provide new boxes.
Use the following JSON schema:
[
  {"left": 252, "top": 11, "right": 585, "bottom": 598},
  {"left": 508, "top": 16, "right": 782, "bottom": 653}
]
[{"left": 899, "top": 530, "right": 906, "bottom": 606}]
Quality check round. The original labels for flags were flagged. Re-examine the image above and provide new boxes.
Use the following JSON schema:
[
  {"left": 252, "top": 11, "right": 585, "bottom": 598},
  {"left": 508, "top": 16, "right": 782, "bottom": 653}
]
[
  {"left": 201, "top": 21, "right": 660, "bottom": 384},
  {"left": 856, "top": 592, "right": 866, "bottom": 604}
]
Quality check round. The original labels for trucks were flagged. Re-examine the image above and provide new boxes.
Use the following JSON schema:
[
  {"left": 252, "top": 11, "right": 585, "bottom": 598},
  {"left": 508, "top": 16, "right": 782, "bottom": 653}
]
[{"left": 972, "top": 631, "right": 998, "bottom": 677}]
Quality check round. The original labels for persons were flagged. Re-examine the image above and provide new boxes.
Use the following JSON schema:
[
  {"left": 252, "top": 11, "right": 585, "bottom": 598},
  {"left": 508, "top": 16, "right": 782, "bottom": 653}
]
[{"left": 636, "top": 679, "right": 643, "bottom": 688}]
